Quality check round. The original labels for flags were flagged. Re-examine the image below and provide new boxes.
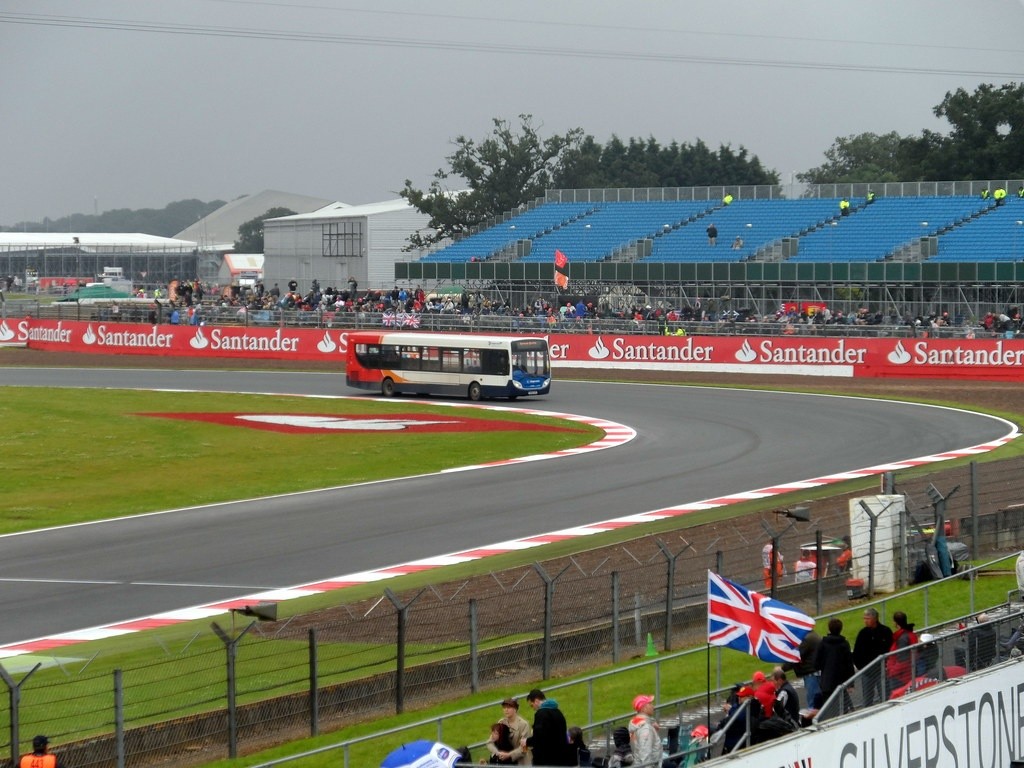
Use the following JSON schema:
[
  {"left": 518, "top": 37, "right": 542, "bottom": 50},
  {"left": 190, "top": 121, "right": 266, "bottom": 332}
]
[
  {"left": 382, "top": 312, "right": 404, "bottom": 327},
  {"left": 707, "top": 570, "right": 816, "bottom": 663},
  {"left": 555, "top": 248, "right": 567, "bottom": 269},
  {"left": 401, "top": 312, "right": 423, "bottom": 328},
  {"left": 553, "top": 270, "right": 569, "bottom": 290}
]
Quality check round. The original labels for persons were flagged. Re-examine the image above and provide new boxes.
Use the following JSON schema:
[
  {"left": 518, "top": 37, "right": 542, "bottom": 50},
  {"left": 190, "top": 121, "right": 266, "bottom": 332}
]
[
  {"left": 16, "top": 735, "right": 63, "bottom": 768},
  {"left": 722, "top": 192, "right": 734, "bottom": 206},
  {"left": 454, "top": 745, "right": 474, "bottom": 768},
  {"left": 980, "top": 186, "right": 1024, "bottom": 207},
  {"left": 0, "top": 286, "right": 5, "bottom": 317},
  {"left": 5, "top": 275, "right": 21, "bottom": 295},
  {"left": 731, "top": 235, "right": 743, "bottom": 249},
  {"left": 608, "top": 695, "right": 663, "bottom": 768},
  {"left": 839, "top": 188, "right": 874, "bottom": 216},
  {"left": 954, "top": 549, "right": 1024, "bottom": 672},
  {"left": 690, "top": 666, "right": 801, "bottom": 756},
  {"left": 706, "top": 223, "right": 718, "bottom": 246},
  {"left": 762, "top": 535, "right": 853, "bottom": 591},
  {"left": 479, "top": 688, "right": 591, "bottom": 768},
  {"left": 771, "top": 607, "right": 968, "bottom": 724},
  {"left": 88, "top": 277, "right": 1024, "bottom": 338}
]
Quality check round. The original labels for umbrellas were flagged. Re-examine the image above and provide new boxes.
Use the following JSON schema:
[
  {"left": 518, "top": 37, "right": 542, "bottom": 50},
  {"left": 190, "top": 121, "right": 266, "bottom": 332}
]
[{"left": 380, "top": 740, "right": 462, "bottom": 768}]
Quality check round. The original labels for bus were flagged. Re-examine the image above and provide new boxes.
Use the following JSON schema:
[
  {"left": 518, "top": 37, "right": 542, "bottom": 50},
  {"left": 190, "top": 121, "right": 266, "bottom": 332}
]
[{"left": 346, "top": 332, "right": 552, "bottom": 401}]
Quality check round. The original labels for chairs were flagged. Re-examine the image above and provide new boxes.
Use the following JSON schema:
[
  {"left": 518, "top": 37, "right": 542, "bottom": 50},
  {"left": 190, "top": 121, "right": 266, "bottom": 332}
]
[{"left": 418, "top": 198, "right": 1024, "bottom": 263}]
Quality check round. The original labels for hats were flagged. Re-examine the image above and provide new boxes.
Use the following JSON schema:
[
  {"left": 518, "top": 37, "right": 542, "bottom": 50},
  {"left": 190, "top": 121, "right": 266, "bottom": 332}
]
[
  {"left": 633, "top": 694, "right": 655, "bottom": 711},
  {"left": 754, "top": 672, "right": 765, "bottom": 682},
  {"left": 920, "top": 634, "right": 935, "bottom": 645},
  {"left": 501, "top": 698, "right": 519, "bottom": 710},
  {"left": 736, "top": 686, "right": 754, "bottom": 697}
]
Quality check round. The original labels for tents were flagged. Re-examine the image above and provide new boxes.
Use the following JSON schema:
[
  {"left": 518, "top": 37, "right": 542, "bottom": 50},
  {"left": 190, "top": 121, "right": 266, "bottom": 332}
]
[{"left": 56, "top": 285, "right": 136, "bottom": 319}]
